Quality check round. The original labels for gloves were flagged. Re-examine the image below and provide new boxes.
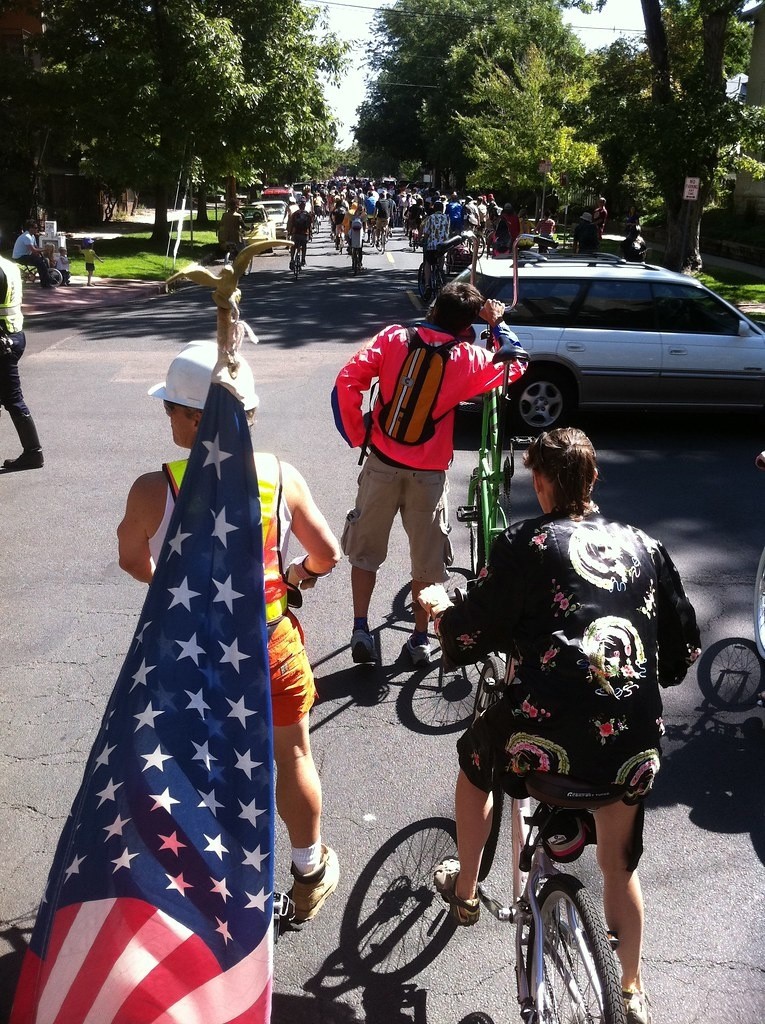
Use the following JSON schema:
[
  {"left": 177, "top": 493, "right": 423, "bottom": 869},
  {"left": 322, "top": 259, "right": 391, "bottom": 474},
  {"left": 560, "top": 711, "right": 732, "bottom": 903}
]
[{"left": 286, "top": 554, "right": 333, "bottom": 590}]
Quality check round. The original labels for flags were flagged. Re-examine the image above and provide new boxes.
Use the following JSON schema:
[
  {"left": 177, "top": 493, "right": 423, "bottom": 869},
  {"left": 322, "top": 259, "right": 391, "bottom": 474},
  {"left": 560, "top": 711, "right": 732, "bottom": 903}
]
[{"left": 9, "top": 350, "right": 275, "bottom": 1024}]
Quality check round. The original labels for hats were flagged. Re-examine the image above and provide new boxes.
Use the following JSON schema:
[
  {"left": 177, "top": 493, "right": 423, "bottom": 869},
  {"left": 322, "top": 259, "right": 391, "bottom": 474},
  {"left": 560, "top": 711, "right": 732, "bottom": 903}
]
[
  {"left": 579, "top": 212, "right": 593, "bottom": 223},
  {"left": 83, "top": 238, "right": 95, "bottom": 245},
  {"left": 503, "top": 202, "right": 512, "bottom": 210},
  {"left": 488, "top": 194, "right": 494, "bottom": 199},
  {"left": 146, "top": 339, "right": 259, "bottom": 411}
]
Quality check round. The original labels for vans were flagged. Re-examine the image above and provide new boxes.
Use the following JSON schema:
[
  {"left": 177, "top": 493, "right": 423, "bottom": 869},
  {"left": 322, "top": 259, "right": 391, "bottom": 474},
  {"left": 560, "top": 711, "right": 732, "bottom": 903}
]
[
  {"left": 261, "top": 185, "right": 295, "bottom": 206},
  {"left": 381, "top": 178, "right": 397, "bottom": 186}
]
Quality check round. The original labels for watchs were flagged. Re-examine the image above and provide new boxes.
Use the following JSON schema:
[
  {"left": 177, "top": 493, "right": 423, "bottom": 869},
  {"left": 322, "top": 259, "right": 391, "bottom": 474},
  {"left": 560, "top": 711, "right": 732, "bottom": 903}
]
[{"left": 426, "top": 599, "right": 443, "bottom": 616}]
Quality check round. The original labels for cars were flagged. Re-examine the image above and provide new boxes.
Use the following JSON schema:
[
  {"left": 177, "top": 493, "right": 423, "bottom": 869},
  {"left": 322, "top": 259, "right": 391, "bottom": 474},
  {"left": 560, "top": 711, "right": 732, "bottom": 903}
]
[
  {"left": 246, "top": 200, "right": 289, "bottom": 240},
  {"left": 292, "top": 183, "right": 314, "bottom": 202},
  {"left": 237, "top": 205, "right": 276, "bottom": 254}
]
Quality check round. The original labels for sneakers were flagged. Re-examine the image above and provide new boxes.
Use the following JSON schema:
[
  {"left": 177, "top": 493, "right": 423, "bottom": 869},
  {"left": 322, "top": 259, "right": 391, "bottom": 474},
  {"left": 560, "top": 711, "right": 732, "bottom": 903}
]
[
  {"left": 3, "top": 449, "right": 45, "bottom": 470},
  {"left": 407, "top": 633, "right": 431, "bottom": 668},
  {"left": 351, "top": 629, "right": 379, "bottom": 664},
  {"left": 289, "top": 843, "right": 341, "bottom": 920}
]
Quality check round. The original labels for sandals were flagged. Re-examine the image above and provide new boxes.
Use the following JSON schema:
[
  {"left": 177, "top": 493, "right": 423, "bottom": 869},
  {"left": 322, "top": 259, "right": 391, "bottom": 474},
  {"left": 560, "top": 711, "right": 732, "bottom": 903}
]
[
  {"left": 432, "top": 855, "right": 482, "bottom": 927},
  {"left": 620, "top": 985, "right": 655, "bottom": 1024}
]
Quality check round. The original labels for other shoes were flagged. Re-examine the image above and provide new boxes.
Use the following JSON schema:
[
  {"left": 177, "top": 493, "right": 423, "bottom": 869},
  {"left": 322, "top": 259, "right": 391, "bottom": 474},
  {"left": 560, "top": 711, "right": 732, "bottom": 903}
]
[
  {"left": 289, "top": 260, "right": 295, "bottom": 271},
  {"left": 40, "top": 279, "right": 59, "bottom": 289},
  {"left": 87, "top": 283, "right": 95, "bottom": 287},
  {"left": 300, "top": 260, "right": 307, "bottom": 267}
]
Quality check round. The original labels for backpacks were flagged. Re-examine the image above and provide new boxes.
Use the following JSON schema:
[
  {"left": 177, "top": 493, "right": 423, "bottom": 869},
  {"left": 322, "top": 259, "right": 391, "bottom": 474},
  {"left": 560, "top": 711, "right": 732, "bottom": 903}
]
[
  {"left": 351, "top": 217, "right": 362, "bottom": 231},
  {"left": 369, "top": 327, "right": 468, "bottom": 445},
  {"left": 493, "top": 216, "right": 512, "bottom": 249}
]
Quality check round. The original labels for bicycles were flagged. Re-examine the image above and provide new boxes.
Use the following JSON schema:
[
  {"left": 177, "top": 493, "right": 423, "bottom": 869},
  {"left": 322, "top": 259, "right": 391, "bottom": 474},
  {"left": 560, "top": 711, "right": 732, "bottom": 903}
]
[
  {"left": 431, "top": 229, "right": 558, "bottom": 583},
  {"left": 15, "top": 247, "right": 64, "bottom": 289},
  {"left": 346, "top": 236, "right": 365, "bottom": 275},
  {"left": 402, "top": 218, "right": 418, "bottom": 252},
  {"left": 414, "top": 240, "right": 445, "bottom": 300},
  {"left": 369, "top": 218, "right": 393, "bottom": 255},
  {"left": 308, "top": 213, "right": 322, "bottom": 242},
  {"left": 443, "top": 588, "right": 627, "bottom": 1024},
  {"left": 290, "top": 233, "right": 312, "bottom": 280},
  {"left": 321, "top": 201, "right": 344, "bottom": 256},
  {"left": 224, "top": 223, "right": 255, "bottom": 276},
  {"left": 474, "top": 224, "right": 496, "bottom": 258},
  {"left": 753, "top": 457, "right": 765, "bottom": 662}
]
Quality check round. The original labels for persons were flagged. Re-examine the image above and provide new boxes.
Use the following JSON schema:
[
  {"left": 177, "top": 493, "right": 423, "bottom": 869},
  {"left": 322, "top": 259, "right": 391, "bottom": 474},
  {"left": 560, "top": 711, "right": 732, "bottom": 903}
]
[
  {"left": 218, "top": 197, "right": 254, "bottom": 276},
  {"left": 331, "top": 282, "right": 532, "bottom": 666},
  {"left": 116, "top": 340, "right": 341, "bottom": 925},
  {"left": 57, "top": 247, "right": 70, "bottom": 286},
  {"left": 79, "top": 238, "right": 104, "bottom": 286},
  {"left": 0, "top": 235, "right": 44, "bottom": 470},
  {"left": 619, "top": 224, "right": 647, "bottom": 262},
  {"left": 41, "top": 243, "right": 58, "bottom": 269},
  {"left": 284, "top": 177, "right": 533, "bottom": 270},
  {"left": 12, "top": 219, "right": 55, "bottom": 289},
  {"left": 572, "top": 212, "right": 601, "bottom": 254},
  {"left": 416, "top": 427, "right": 703, "bottom": 1024},
  {"left": 418, "top": 202, "right": 451, "bottom": 299},
  {"left": 531, "top": 211, "right": 555, "bottom": 253},
  {"left": 593, "top": 197, "right": 608, "bottom": 240}
]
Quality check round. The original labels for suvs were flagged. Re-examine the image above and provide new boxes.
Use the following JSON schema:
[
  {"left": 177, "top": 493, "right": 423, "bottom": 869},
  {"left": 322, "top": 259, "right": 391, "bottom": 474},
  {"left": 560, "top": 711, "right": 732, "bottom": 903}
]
[{"left": 424, "top": 251, "right": 765, "bottom": 438}]
